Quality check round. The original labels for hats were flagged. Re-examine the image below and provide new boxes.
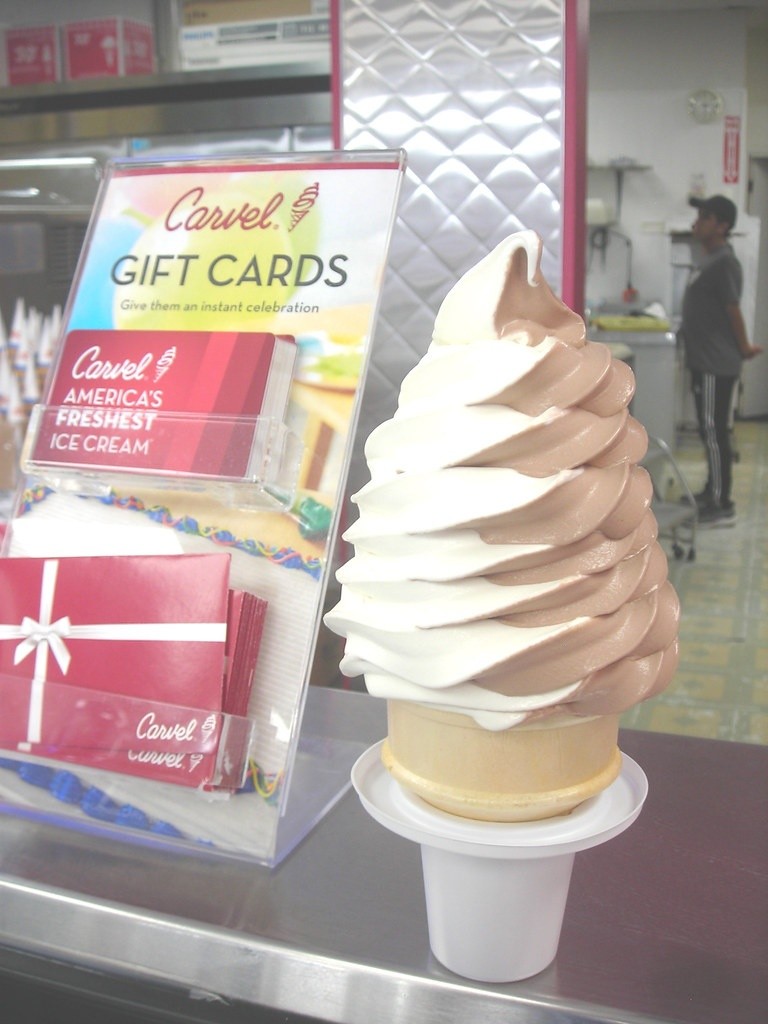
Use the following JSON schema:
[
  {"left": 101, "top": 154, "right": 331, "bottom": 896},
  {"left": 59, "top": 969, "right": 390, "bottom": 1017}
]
[{"left": 688, "top": 196, "right": 736, "bottom": 227}]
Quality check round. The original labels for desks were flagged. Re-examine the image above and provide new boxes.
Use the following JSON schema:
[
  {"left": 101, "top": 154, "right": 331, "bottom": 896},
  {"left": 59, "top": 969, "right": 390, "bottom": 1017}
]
[{"left": 0, "top": 632, "right": 768, "bottom": 1024}]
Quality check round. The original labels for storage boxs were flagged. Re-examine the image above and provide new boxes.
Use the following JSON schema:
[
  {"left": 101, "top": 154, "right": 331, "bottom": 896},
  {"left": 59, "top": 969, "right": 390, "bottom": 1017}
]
[
  {"left": 169, "top": 0, "right": 333, "bottom": 72},
  {"left": 63, "top": 15, "right": 149, "bottom": 82},
  {"left": 6, "top": 21, "right": 58, "bottom": 88}
]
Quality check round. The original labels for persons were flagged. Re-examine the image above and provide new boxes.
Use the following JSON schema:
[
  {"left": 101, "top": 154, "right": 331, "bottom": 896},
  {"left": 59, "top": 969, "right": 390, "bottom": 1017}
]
[{"left": 680, "top": 192, "right": 763, "bottom": 531}]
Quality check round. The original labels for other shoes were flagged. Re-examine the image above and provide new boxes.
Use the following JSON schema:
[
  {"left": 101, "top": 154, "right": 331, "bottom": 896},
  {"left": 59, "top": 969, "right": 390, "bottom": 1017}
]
[
  {"left": 681, "top": 508, "right": 737, "bottom": 529},
  {"left": 680, "top": 494, "right": 709, "bottom": 507}
]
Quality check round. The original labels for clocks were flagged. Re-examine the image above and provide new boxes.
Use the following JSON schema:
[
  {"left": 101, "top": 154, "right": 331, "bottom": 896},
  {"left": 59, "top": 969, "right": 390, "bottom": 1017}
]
[{"left": 686, "top": 90, "right": 723, "bottom": 123}]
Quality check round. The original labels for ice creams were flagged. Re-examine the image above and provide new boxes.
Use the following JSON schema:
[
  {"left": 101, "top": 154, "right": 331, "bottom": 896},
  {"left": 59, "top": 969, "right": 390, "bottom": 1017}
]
[{"left": 324, "top": 231, "right": 684, "bottom": 819}]
